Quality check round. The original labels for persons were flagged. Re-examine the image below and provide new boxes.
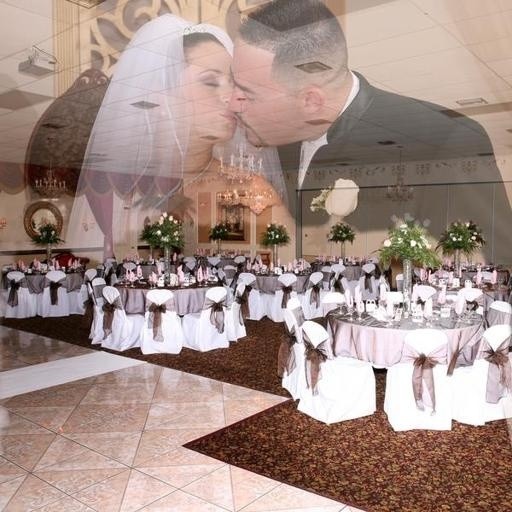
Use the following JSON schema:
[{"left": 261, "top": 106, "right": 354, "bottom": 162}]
[
  {"left": 228, "top": 0, "right": 512, "bottom": 307},
  {"left": 64, "top": 14, "right": 301, "bottom": 269}
]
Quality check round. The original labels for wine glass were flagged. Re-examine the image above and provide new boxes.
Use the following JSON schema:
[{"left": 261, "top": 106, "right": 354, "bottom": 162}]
[
  {"left": 347, "top": 302, "right": 365, "bottom": 322},
  {"left": 197, "top": 273, "right": 209, "bottom": 287},
  {"left": 148, "top": 279, "right": 157, "bottom": 289},
  {"left": 456, "top": 304, "right": 465, "bottom": 322}
]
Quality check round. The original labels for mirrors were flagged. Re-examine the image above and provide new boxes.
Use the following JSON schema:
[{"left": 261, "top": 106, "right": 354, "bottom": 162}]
[{"left": 23, "top": 201, "right": 63, "bottom": 241}]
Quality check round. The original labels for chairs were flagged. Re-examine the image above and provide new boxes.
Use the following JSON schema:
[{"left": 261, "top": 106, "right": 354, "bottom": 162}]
[{"left": 0, "top": 251, "right": 512, "bottom": 431}]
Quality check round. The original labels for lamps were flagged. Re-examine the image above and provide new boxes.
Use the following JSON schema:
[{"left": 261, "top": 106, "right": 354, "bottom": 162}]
[
  {"left": 386, "top": 150, "right": 414, "bottom": 201},
  {"left": 35, "top": 157, "right": 68, "bottom": 198},
  {"left": 217, "top": 137, "right": 282, "bottom": 215}
]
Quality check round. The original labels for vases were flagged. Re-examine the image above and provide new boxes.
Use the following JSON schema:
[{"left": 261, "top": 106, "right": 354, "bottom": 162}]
[
  {"left": 164, "top": 245, "right": 172, "bottom": 284},
  {"left": 273, "top": 243, "right": 277, "bottom": 267},
  {"left": 341, "top": 243, "right": 345, "bottom": 258},
  {"left": 454, "top": 247, "right": 461, "bottom": 277},
  {"left": 47, "top": 242, "right": 52, "bottom": 269},
  {"left": 218, "top": 238, "right": 221, "bottom": 254}
]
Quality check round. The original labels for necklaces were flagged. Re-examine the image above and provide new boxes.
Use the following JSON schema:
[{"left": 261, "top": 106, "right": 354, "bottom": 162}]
[{"left": 180, "top": 155, "right": 214, "bottom": 187}]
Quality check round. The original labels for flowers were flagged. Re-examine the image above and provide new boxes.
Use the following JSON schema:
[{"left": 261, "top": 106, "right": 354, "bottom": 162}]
[
  {"left": 309, "top": 177, "right": 360, "bottom": 218},
  {"left": 208, "top": 222, "right": 228, "bottom": 240},
  {"left": 139, "top": 212, "right": 183, "bottom": 249},
  {"left": 435, "top": 219, "right": 486, "bottom": 258},
  {"left": 33, "top": 224, "right": 64, "bottom": 246},
  {"left": 260, "top": 223, "right": 291, "bottom": 246},
  {"left": 369, "top": 213, "right": 443, "bottom": 270},
  {"left": 326, "top": 222, "right": 356, "bottom": 246}
]
